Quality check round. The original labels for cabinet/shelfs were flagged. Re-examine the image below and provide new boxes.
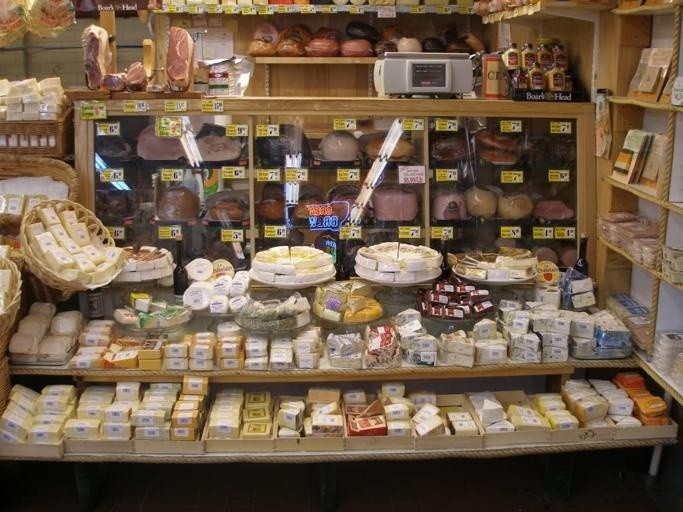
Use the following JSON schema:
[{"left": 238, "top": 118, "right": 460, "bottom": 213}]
[
  {"left": 0, "top": 0, "right": 595, "bottom": 88},
  {"left": 1, "top": 92, "right": 678, "bottom": 476},
  {"left": 596, "top": 1, "right": 682, "bottom": 477},
  {"left": 0, "top": 76, "right": 75, "bottom": 416}
]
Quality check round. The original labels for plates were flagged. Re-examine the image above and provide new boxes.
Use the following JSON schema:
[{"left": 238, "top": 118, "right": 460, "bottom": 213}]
[
  {"left": 247, "top": 267, "right": 338, "bottom": 291},
  {"left": 450, "top": 264, "right": 538, "bottom": 288},
  {"left": 351, "top": 267, "right": 443, "bottom": 288}
]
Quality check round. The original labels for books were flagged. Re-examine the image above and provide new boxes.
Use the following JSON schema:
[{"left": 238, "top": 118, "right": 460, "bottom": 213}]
[{"left": 608, "top": 47, "right": 674, "bottom": 189}]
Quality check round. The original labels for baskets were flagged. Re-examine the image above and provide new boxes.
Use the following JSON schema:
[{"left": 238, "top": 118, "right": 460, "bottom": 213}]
[
  {"left": 20, "top": 198, "right": 117, "bottom": 291},
  {"left": 0, "top": 151, "right": 81, "bottom": 228},
  {"left": 0, "top": 254, "right": 23, "bottom": 362},
  {"left": 0, "top": 101, "right": 76, "bottom": 159}
]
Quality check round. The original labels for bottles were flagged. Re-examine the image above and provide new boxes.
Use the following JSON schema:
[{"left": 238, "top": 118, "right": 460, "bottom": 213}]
[
  {"left": 172, "top": 232, "right": 189, "bottom": 295},
  {"left": 502, "top": 41, "right": 570, "bottom": 91},
  {"left": 87, "top": 286, "right": 105, "bottom": 320}
]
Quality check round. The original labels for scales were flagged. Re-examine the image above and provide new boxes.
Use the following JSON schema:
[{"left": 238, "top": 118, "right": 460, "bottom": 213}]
[{"left": 372, "top": 50, "right": 473, "bottom": 100}]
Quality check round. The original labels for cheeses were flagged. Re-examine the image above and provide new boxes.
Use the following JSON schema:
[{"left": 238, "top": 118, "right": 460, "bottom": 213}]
[
  {"left": 0, "top": 130, "right": 683, "bottom": 444},
  {"left": 0, "top": 77, "right": 64, "bottom": 121}
]
